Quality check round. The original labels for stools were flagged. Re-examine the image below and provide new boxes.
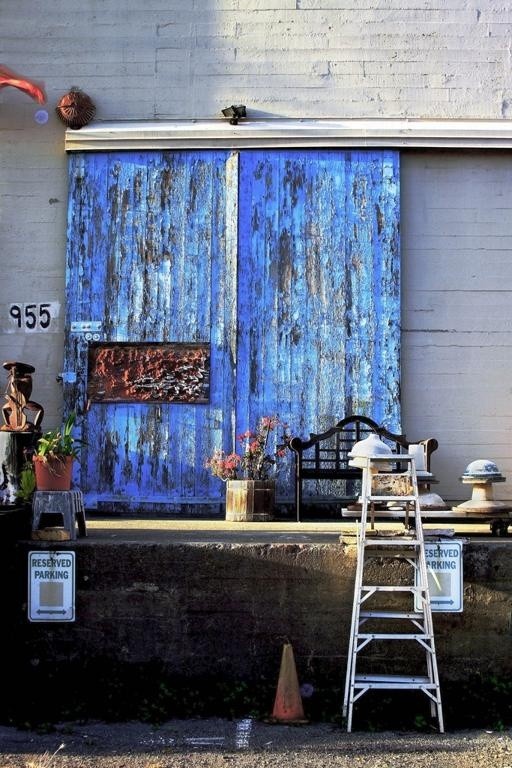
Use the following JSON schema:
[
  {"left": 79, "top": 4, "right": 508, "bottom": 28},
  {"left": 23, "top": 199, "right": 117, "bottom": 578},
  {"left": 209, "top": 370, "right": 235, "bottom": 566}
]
[{"left": 30, "top": 491, "right": 88, "bottom": 541}]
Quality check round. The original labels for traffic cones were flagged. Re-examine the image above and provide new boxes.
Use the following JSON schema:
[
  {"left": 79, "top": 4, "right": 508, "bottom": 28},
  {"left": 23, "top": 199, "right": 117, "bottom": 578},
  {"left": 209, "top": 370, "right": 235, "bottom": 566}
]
[{"left": 263, "top": 642, "right": 311, "bottom": 727}]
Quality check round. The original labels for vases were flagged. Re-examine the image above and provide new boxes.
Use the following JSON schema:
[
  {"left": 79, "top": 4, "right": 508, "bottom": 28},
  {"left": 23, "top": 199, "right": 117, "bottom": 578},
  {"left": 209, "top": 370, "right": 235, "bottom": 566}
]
[{"left": 226, "top": 479, "right": 276, "bottom": 522}]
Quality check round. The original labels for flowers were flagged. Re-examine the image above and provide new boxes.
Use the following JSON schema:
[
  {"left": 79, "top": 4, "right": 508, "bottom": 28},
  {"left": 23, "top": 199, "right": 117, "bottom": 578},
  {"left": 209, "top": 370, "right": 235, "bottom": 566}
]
[{"left": 205, "top": 415, "right": 293, "bottom": 482}]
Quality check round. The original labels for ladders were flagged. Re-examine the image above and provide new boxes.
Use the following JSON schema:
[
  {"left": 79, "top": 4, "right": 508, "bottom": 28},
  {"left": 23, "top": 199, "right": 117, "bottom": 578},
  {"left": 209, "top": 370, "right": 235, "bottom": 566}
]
[{"left": 342, "top": 453, "right": 445, "bottom": 734}]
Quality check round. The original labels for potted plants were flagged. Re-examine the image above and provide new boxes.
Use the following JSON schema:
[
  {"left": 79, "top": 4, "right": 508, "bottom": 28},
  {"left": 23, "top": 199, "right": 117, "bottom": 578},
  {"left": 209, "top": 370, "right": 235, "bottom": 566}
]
[{"left": 32, "top": 411, "right": 90, "bottom": 491}]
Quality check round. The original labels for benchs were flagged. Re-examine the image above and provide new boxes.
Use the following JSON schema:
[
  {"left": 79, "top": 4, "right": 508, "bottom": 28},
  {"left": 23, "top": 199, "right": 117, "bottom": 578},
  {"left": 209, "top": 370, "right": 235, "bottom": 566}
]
[{"left": 288, "top": 415, "right": 438, "bottom": 522}]
